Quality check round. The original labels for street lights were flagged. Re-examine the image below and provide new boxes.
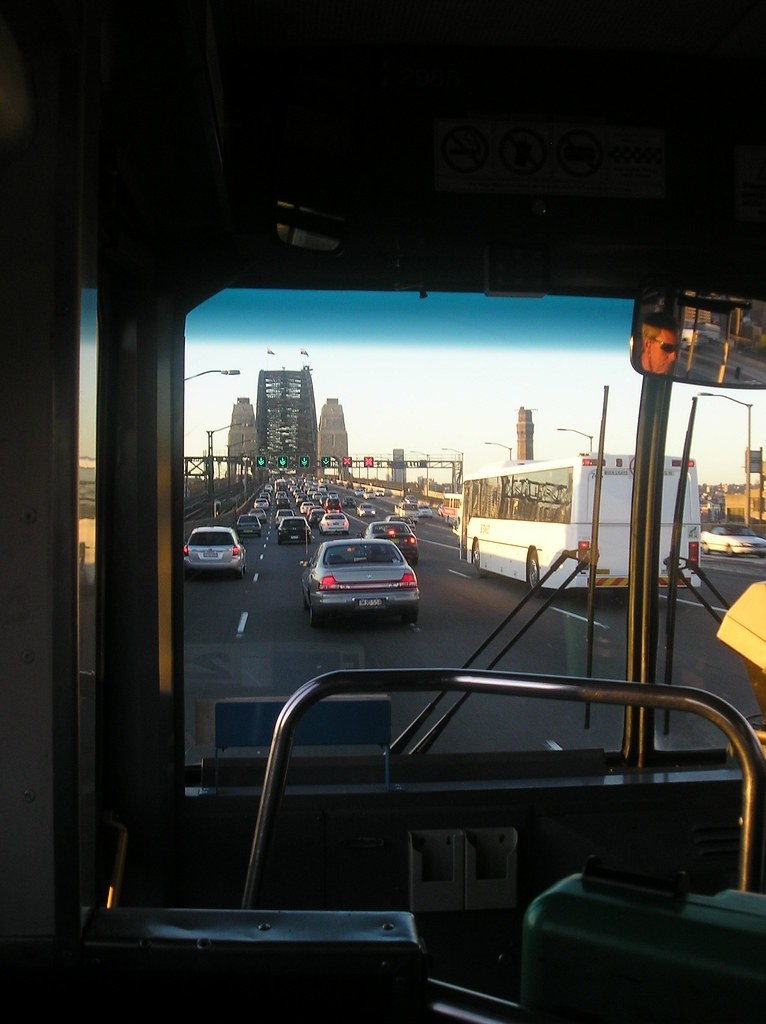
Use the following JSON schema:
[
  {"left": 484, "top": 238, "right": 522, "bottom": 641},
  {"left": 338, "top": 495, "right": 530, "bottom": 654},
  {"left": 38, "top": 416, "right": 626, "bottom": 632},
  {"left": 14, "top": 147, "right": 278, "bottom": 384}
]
[
  {"left": 207, "top": 423, "right": 253, "bottom": 501},
  {"left": 558, "top": 429, "right": 593, "bottom": 452},
  {"left": 485, "top": 442, "right": 512, "bottom": 461},
  {"left": 442, "top": 447, "right": 465, "bottom": 493},
  {"left": 410, "top": 450, "right": 429, "bottom": 497},
  {"left": 698, "top": 392, "right": 754, "bottom": 528}
]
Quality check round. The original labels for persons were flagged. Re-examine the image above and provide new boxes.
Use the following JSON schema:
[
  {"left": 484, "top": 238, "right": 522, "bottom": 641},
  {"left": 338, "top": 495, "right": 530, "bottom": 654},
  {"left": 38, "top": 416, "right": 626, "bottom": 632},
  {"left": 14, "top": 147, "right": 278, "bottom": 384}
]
[{"left": 640, "top": 312, "right": 680, "bottom": 374}]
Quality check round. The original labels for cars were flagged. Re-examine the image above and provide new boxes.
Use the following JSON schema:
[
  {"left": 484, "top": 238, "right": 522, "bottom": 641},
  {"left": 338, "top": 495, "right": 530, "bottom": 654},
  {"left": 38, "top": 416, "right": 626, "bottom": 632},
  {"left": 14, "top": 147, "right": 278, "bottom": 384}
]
[
  {"left": 357, "top": 521, "right": 418, "bottom": 566},
  {"left": 299, "top": 537, "right": 420, "bottom": 628},
  {"left": 701, "top": 525, "right": 766, "bottom": 558},
  {"left": 383, "top": 515, "right": 416, "bottom": 533},
  {"left": 405, "top": 494, "right": 433, "bottom": 519},
  {"left": 236, "top": 477, "right": 386, "bottom": 537}
]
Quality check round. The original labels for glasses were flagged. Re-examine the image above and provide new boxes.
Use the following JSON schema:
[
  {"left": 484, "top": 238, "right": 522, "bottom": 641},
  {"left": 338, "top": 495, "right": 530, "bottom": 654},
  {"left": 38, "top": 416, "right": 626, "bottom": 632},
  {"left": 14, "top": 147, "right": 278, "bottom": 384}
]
[{"left": 653, "top": 337, "right": 678, "bottom": 353}]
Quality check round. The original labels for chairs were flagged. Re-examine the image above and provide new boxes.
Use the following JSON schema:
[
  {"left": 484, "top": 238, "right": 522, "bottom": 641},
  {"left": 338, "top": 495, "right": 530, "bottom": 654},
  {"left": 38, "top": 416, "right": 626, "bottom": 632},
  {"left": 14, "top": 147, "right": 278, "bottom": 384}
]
[{"left": 80, "top": 902, "right": 428, "bottom": 1024}]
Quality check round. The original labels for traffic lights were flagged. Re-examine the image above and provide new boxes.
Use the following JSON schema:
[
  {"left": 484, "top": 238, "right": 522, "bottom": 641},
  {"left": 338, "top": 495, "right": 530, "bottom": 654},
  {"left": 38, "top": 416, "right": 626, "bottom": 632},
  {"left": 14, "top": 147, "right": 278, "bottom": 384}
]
[{"left": 257, "top": 456, "right": 374, "bottom": 468}]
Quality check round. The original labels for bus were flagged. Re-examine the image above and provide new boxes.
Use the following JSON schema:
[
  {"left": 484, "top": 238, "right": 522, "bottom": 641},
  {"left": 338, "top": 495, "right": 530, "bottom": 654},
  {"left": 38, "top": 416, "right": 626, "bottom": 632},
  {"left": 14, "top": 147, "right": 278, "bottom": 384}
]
[
  {"left": 442, "top": 493, "right": 463, "bottom": 526},
  {"left": 274, "top": 479, "right": 286, "bottom": 493},
  {"left": 459, "top": 453, "right": 702, "bottom": 598}
]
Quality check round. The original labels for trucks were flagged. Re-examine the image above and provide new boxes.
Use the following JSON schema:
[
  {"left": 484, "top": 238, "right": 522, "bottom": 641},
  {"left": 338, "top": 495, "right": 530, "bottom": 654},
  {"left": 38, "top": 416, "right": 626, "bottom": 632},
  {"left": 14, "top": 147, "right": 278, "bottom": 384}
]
[{"left": 395, "top": 504, "right": 421, "bottom": 524}]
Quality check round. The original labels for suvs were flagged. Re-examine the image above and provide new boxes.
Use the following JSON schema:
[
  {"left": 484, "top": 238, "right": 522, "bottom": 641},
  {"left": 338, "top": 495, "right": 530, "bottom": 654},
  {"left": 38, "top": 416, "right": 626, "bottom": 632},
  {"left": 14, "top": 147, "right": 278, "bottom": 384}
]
[
  {"left": 276, "top": 516, "right": 312, "bottom": 545},
  {"left": 183, "top": 526, "right": 247, "bottom": 579},
  {"left": 629, "top": 317, "right": 699, "bottom": 357}
]
